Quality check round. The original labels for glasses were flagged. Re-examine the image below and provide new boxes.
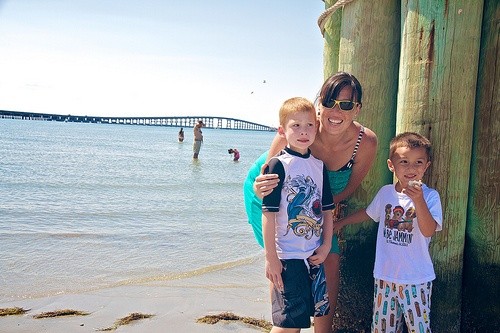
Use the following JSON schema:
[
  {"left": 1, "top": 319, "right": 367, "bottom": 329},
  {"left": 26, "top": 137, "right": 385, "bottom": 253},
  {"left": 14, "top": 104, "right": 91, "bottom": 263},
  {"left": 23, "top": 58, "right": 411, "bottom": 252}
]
[{"left": 321, "top": 97, "right": 360, "bottom": 111}]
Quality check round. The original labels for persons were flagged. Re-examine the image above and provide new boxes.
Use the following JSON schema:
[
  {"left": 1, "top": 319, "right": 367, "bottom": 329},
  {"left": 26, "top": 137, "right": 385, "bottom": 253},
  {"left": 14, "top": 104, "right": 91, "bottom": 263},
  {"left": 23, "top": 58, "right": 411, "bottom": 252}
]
[
  {"left": 333, "top": 132, "right": 444, "bottom": 333},
  {"left": 254, "top": 71, "right": 377, "bottom": 333},
  {"left": 260, "top": 98, "right": 333, "bottom": 333},
  {"left": 178, "top": 127, "right": 184, "bottom": 142},
  {"left": 192, "top": 120, "right": 203, "bottom": 159},
  {"left": 228, "top": 148, "right": 240, "bottom": 161}
]
